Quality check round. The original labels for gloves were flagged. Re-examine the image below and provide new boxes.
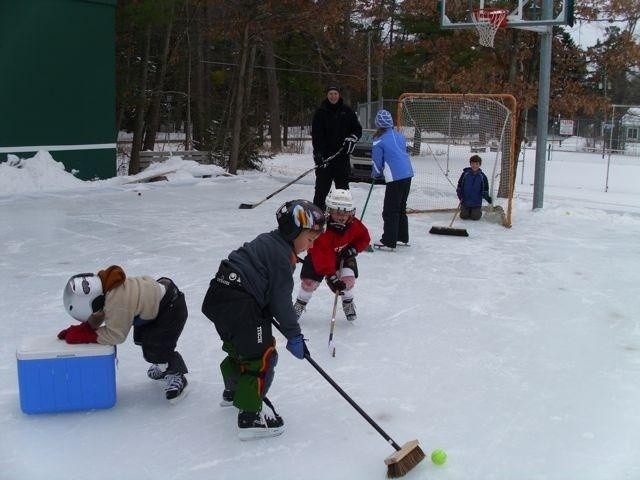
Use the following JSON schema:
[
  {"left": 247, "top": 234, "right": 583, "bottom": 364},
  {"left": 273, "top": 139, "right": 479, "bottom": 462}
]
[
  {"left": 327, "top": 273, "right": 346, "bottom": 292},
  {"left": 338, "top": 245, "right": 359, "bottom": 259},
  {"left": 313, "top": 152, "right": 329, "bottom": 170},
  {"left": 341, "top": 134, "right": 359, "bottom": 154},
  {"left": 58, "top": 324, "right": 97, "bottom": 345},
  {"left": 286, "top": 336, "right": 310, "bottom": 360}
]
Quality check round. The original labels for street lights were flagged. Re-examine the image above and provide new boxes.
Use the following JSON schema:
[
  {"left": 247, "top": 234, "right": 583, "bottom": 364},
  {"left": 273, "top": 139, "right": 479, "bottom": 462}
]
[{"left": 598, "top": 63, "right": 612, "bottom": 123}]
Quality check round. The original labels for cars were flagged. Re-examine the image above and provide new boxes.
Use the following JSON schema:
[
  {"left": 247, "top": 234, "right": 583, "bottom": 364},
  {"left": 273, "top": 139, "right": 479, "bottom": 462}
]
[
  {"left": 469, "top": 141, "right": 486, "bottom": 153},
  {"left": 347, "top": 128, "right": 386, "bottom": 185},
  {"left": 490, "top": 140, "right": 498, "bottom": 152}
]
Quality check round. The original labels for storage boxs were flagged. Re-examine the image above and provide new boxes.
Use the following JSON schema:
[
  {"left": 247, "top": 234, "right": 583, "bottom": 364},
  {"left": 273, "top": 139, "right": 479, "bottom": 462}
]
[{"left": 13, "top": 329, "right": 118, "bottom": 417}]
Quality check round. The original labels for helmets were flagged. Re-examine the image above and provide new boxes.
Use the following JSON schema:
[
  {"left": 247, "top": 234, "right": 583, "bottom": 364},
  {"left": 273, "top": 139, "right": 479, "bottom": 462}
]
[
  {"left": 276, "top": 199, "right": 325, "bottom": 239},
  {"left": 63, "top": 272, "right": 106, "bottom": 323},
  {"left": 325, "top": 189, "right": 356, "bottom": 230}
]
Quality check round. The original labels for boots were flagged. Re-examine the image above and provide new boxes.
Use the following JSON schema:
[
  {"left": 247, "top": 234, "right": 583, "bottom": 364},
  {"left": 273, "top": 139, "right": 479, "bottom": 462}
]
[
  {"left": 237, "top": 409, "right": 286, "bottom": 441},
  {"left": 294, "top": 302, "right": 306, "bottom": 321},
  {"left": 149, "top": 363, "right": 190, "bottom": 404},
  {"left": 219, "top": 389, "right": 240, "bottom": 406},
  {"left": 373, "top": 238, "right": 410, "bottom": 252},
  {"left": 341, "top": 298, "right": 358, "bottom": 325}
]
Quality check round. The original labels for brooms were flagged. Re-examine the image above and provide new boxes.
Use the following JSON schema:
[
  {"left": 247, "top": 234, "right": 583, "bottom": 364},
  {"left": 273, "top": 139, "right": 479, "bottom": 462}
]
[
  {"left": 430, "top": 201, "right": 469, "bottom": 237},
  {"left": 269, "top": 317, "right": 426, "bottom": 480},
  {"left": 359, "top": 181, "right": 374, "bottom": 253}
]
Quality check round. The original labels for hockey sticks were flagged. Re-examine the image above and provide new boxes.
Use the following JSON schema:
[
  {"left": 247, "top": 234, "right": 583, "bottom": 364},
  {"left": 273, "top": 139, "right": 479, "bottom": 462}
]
[
  {"left": 239, "top": 147, "right": 342, "bottom": 208},
  {"left": 328, "top": 258, "right": 345, "bottom": 357}
]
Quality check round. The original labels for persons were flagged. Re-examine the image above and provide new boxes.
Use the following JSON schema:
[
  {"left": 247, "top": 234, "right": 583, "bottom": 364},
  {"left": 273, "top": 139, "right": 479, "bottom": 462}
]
[
  {"left": 371, "top": 108, "right": 416, "bottom": 249},
  {"left": 456, "top": 155, "right": 491, "bottom": 220},
  {"left": 200, "top": 197, "right": 328, "bottom": 432},
  {"left": 309, "top": 81, "right": 364, "bottom": 213},
  {"left": 292, "top": 187, "right": 372, "bottom": 324},
  {"left": 57, "top": 264, "right": 188, "bottom": 399}
]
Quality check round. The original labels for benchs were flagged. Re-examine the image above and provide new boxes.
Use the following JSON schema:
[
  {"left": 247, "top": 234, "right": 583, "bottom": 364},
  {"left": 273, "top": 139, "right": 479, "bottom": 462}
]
[
  {"left": 470, "top": 141, "right": 487, "bottom": 153},
  {"left": 490, "top": 141, "right": 500, "bottom": 152}
]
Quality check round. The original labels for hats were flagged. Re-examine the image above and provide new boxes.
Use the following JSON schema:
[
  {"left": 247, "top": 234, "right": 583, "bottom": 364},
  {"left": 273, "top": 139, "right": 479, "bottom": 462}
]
[
  {"left": 375, "top": 109, "right": 393, "bottom": 128},
  {"left": 326, "top": 84, "right": 341, "bottom": 95}
]
